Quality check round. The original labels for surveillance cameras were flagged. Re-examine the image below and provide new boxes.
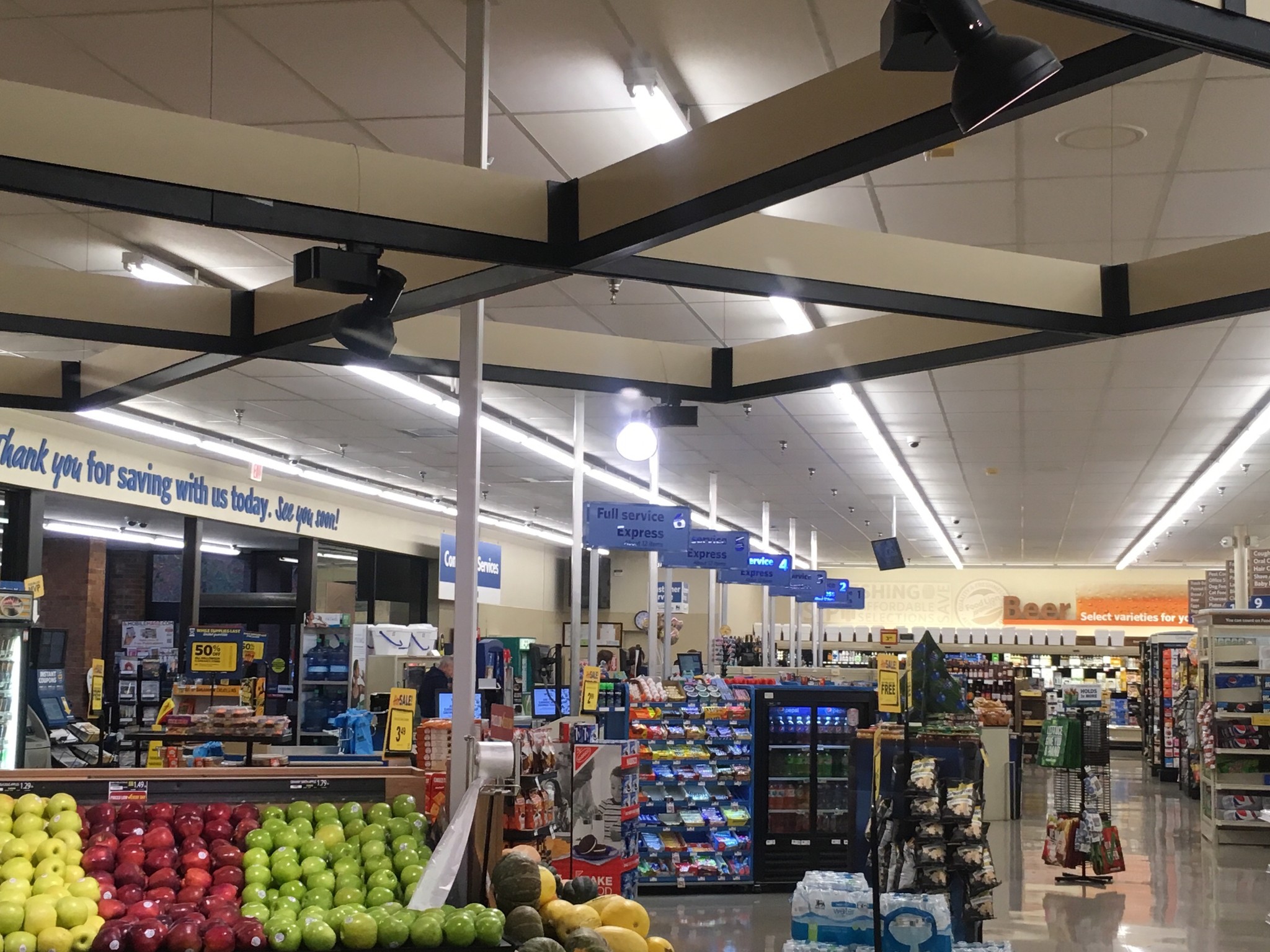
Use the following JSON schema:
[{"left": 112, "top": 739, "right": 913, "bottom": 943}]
[
  {"left": 288, "top": 455, "right": 302, "bottom": 466},
  {"left": 954, "top": 532, "right": 963, "bottom": 538},
  {"left": 906, "top": 436, "right": 921, "bottom": 449},
  {"left": 950, "top": 516, "right": 960, "bottom": 524},
  {"left": 524, "top": 520, "right": 533, "bottom": 527},
  {"left": 124, "top": 514, "right": 137, "bottom": 526},
  {"left": 962, "top": 544, "right": 971, "bottom": 550},
  {"left": 137, "top": 514, "right": 150, "bottom": 528},
  {"left": 432, "top": 495, "right": 443, "bottom": 504}
]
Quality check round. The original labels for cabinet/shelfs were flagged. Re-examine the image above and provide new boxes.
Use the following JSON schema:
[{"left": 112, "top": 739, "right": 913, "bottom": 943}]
[
  {"left": 124, "top": 732, "right": 297, "bottom": 771},
  {"left": 1011, "top": 664, "right": 1140, "bottom": 748},
  {"left": 502, "top": 771, "right": 561, "bottom": 864},
  {"left": 1199, "top": 606, "right": 1270, "bottom": 845},
  {"left": 628, "top": 685, "right": 757, "bottom": 886}
]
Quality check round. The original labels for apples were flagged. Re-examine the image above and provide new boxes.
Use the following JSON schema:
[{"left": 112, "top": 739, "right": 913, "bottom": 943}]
[{"left": 0, "top": 789, "right": 507, "bottom": 952}]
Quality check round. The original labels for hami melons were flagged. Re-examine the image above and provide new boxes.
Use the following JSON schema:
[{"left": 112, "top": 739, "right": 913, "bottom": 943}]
[{"left": 538, "top": 866, "right": 676, "bottom": 952}]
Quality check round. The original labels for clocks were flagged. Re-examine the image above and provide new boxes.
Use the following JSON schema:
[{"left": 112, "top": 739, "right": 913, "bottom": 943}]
[{"left": 634, "top": 610, "right": 649, "bottom": 630}]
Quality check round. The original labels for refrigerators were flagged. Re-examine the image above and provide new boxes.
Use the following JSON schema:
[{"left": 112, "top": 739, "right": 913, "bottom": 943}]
[
  {"left": 0, "top": 589, "right": 33, "bottom": 769},
  {"left": 753, "top": 688, "right": 875, "bottom": 885}
]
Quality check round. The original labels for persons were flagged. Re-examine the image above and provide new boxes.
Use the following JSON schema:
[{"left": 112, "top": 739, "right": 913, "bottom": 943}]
[
  {"left": 596, "top": 649, "right": 613, "bottom": 672},
  {"left": 416, "top": 655, "right": 454, "bottom": 718},
  {"left": 304, "top": 609, "right": 329, "bottom": 628},
  {"left": 572, "top": 757, "right": 621, "bottom": 841},
  {"left": 351, "top": 660, "right": 366, "bottom": 708}
]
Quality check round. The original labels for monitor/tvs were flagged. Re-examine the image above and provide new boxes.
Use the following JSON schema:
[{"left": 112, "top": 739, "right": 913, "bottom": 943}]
[
  {"left": 529, "top": 643, "right": 554, "bottom": 684},
  {"left": 677, "top": 652, "right": 704, "bottom": 675},
  {"left": 29, "top": 627, "right": 69, "bottom": 669},
  {"left": 434, "top": 688, "right": 485, "bottom": 719},
  {"left": 532, "top": 685, "right": 570, "bottom": 722},
  {"left": 870, "top": 537, "right": 906, "bottom": 570}
]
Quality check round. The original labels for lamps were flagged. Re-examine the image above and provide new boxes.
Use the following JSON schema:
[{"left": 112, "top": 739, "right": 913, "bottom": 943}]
[{"left": 43, "top": 0, "right": 1270, "bottom": 572}]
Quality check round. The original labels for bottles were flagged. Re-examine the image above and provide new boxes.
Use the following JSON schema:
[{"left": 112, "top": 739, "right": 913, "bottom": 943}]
[
  {"left": 767, "top": 780, "right": 849, "bottom": 833},
  {"left": 304, "top": 688, "right": 347, "bottom": 732},
  {"left": 767, "top": 713, "right": 858, "bottom": 745},
  {"left": 767, "top": 747, "right": 851, "bottom": 778},
  {"left": 307, "top": 633, "right": 349, "bottom": 681}
]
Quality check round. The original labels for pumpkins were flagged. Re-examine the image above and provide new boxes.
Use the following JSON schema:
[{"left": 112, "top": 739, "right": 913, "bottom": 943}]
[{"left": 492, "top": 843, "right": 613, "bottom": 952}]
